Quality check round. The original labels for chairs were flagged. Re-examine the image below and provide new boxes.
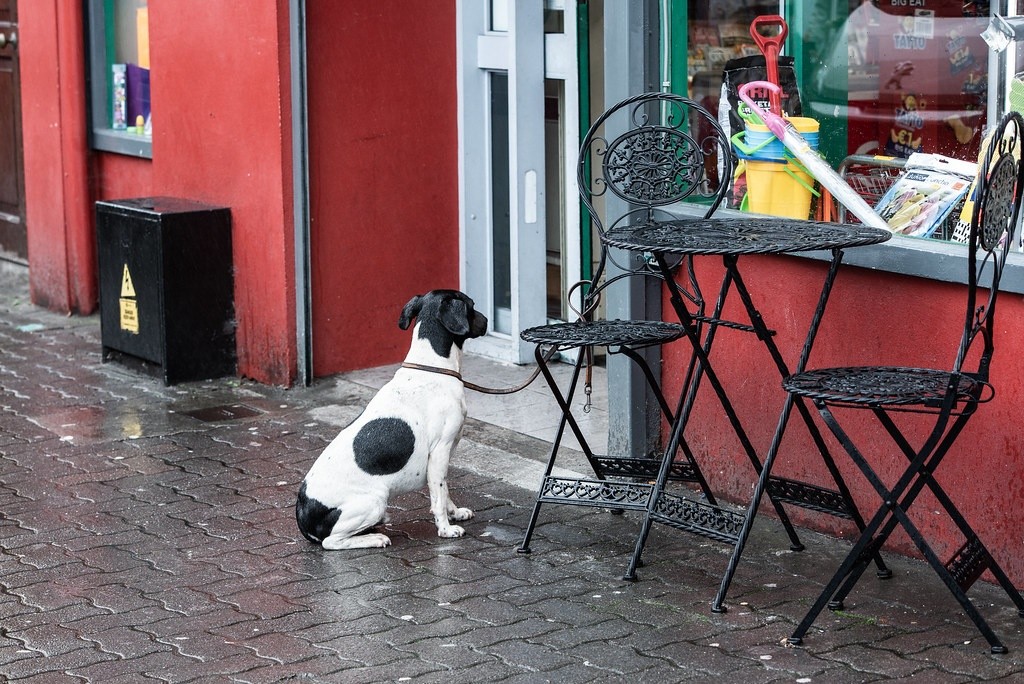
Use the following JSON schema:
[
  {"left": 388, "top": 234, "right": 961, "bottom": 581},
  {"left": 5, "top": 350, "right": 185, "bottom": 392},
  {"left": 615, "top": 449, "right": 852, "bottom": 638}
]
[
  {"left": 782, "top": 111, "right": 1024, "bottom": 655},
  {"left": 517, "top": 90, "right": 732, "bottom": 569}
]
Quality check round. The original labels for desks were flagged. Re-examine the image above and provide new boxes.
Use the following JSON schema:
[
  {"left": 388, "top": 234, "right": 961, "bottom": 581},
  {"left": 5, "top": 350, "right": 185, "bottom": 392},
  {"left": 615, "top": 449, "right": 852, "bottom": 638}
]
[{"left": 600, "top": 217, "right": 892, "bottom": 616}]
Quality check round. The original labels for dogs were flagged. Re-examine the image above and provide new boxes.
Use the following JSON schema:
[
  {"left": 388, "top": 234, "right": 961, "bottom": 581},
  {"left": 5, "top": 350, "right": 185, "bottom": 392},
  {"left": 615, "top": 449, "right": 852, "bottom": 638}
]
[{"left": 295, "top": 288, "right": 489, "bottom": 551}]
